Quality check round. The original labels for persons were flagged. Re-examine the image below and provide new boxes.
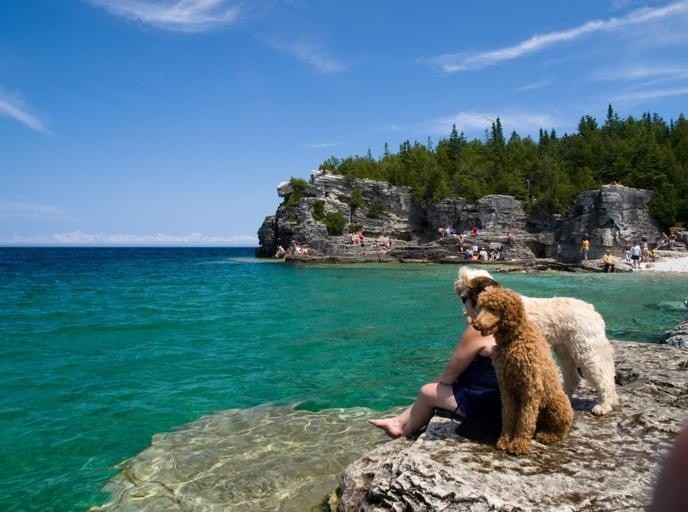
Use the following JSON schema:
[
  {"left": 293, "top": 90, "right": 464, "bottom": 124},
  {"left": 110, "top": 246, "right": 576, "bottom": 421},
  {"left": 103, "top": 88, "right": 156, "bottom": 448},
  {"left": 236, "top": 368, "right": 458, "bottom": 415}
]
[
  {"left": 603, "top": 250, "right": 614, "bottom": 272},
  {"left": 622, "top": 238, "right": 648, "bottom": 269},
  {"left": 364, "top": 274, "right": 504, "bottom": 443},
  {"left": 654, "top": 231, "right": 677, "bottom": 250},
  {"left": 529, "top": 195, "right": 536, "bottom": 206},
  {"left": 437, "top": 224, "right": 500, "bottom": 261},
  {"left": 351, "top": 232, "right": 364, "bottom": 244},
  {"left": 274, "top": 240, "right": 309, "bottom": 258},
  {"left": 383, "top": 235, "right": 393, "bottom": 249},
  {"left": 580, "top": 237, "right": 590, "bottom": 261}
]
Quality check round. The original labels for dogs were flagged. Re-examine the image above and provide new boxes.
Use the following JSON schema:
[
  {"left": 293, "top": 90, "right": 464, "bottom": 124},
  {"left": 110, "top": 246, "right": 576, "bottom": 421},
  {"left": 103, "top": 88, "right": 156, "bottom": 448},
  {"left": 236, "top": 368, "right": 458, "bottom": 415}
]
[
  {"left": 472, "top": 285, "right": 575, "bottom": 459},
  {"left": 453, "top": 265, "right": 620, "bottom": 417}
]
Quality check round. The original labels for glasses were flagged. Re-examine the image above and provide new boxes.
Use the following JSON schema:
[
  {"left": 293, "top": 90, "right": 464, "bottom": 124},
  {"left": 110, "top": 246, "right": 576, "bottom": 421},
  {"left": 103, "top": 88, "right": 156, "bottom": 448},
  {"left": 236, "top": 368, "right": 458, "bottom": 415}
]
[{"left": 461, "top": 296, "right": 472, "bottom": 303}]
